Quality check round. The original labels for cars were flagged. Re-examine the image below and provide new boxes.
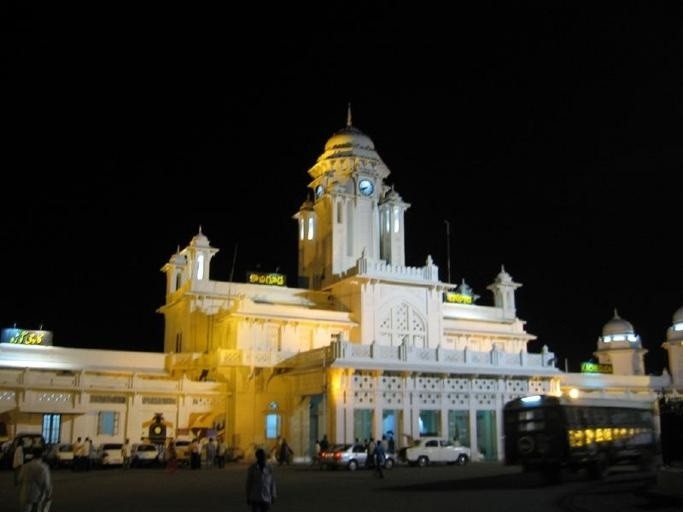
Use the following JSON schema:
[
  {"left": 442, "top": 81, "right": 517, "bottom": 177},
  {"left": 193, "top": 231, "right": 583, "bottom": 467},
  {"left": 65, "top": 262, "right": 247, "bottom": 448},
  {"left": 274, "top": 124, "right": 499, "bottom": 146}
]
[
  {"left": 1, "top": 433, "right": 197, "bottom": 470},
  {"left": 400, "top": 435, "right": 471, "bottom": 467},
  {"left": 321, "top": 443, "right": 400, "bottom": 470}
]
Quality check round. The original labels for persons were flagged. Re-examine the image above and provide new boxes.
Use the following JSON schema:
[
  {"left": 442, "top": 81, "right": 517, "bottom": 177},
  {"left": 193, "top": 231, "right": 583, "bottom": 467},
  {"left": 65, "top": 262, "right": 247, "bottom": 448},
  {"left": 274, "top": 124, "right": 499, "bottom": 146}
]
[
  {"left": 355, "top": 432, "right": 395, "bottom": 478},
  {"left": 121, "top": 438, "right": 133, "bottom": 470},
  {"left": 18, "top": 447, "right": 54, "bottom": 512},
  {"left": 12, "top": 441, "right": 23, "bottom": 484},
  {"left": 73, "top": 437, "right": 96, "bottom": 473},
  {"left": 319, "top": 434, "right": 329, "bottom": 451},
  {"left": 309, "top": 439, "right": 323, "bottom": 469},
  {"left": 245, "top": 449, "right": 277, "bottom": 512},
  {"left": 273, "top": 436, "right": 282, "bottom": 463},
  {"left": 189, "top": 438, "right": 226, "bottom": 470},
  {"left": 139, "top": 437, "right": 146, "bottom": 444},
  {"left": 279, "top": 439, "right": 291, "bottom": 466}
]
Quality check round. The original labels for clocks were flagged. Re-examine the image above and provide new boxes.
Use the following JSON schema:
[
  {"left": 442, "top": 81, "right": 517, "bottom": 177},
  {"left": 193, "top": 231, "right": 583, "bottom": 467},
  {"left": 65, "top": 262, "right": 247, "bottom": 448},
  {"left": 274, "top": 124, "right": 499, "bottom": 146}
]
[
  {"left": 359, "top": 179, "right": 373, "bottom": 196},
  {"left": 315, "top": 184, "right": 324, "bottom": 200}
]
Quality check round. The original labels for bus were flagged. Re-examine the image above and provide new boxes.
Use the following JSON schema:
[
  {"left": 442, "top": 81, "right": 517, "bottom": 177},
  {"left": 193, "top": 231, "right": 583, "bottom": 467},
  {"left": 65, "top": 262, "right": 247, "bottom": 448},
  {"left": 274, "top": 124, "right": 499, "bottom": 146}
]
[{"left": 503, "top": 391, "right": 660, "bottom": 475}]
